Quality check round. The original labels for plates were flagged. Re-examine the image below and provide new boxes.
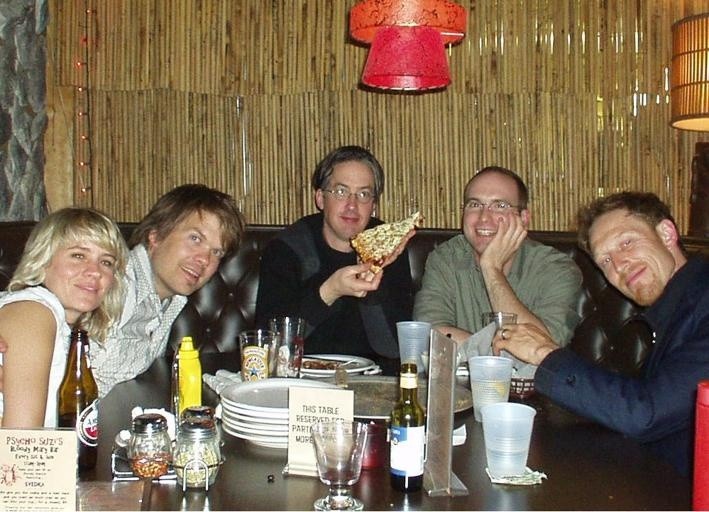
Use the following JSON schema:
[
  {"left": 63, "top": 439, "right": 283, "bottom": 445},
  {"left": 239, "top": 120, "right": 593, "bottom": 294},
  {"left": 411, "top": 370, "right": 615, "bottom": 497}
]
[
  {"left": 219, "top": 376, "right": 338, "bottom": 451},
  {"left": 299, "top": 353, "right": 378, "bottom": 377},
  {"left": 338, "top": 375, "right": 473, "bottom": 420}
]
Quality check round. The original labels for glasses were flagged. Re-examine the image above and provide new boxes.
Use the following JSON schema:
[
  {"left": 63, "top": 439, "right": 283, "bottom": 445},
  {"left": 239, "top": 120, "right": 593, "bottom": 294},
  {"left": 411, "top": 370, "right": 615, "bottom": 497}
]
[
  {"left": 458, "top": 200, "right": 524, "bottom": 213},
  {"left": 320, "top": 186, "right": 377, "bottom": 204}
]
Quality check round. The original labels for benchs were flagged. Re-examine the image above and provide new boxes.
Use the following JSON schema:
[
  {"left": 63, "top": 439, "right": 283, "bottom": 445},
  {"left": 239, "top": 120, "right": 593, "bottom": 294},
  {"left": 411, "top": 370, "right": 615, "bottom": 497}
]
[{"left": 0, "top": 217, "right": 709, "bottom": 394}]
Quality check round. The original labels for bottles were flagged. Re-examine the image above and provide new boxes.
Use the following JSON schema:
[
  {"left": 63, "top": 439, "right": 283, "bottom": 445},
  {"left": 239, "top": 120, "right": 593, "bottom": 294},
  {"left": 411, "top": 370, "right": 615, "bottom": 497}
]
[
  {"left": 57, "top": 329, "right": 100, "bottom": 473},
  {"left": 129, "top": 404, "right": 221, "bottom": 489},
  {"left": 388, "top": 361, "right": 425, "bottom": 488},
  {"left": 175, "top": 335, "right": 201, "bottom": 416}
]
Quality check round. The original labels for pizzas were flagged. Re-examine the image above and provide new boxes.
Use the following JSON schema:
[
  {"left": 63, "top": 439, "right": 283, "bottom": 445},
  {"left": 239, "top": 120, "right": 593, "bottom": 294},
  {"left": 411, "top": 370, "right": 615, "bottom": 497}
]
[{"left": 349, "top": 210, "right": 422, "bottom": 281}]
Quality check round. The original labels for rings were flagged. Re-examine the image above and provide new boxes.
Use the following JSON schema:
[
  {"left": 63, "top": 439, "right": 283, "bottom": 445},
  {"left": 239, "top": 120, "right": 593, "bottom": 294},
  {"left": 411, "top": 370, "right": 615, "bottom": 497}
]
[{"left": 502, "top": 328, "right": 509, "bottom": 340}]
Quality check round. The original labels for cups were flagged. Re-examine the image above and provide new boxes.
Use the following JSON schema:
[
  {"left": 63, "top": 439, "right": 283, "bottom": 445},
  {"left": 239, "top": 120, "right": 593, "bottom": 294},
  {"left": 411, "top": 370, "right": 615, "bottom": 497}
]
[
  {"left": 467, "top": 354, "right": 515, "bottom": 420},
  {"left": 482, "top": 310, "right": 518, "bottom": 333},
  {"left": 479, "top": 402, "right": 537, "bottom": 479},
  {"left": 395, "top": 320, "right": 431, "bottom": 372},
  {"left": 238, "top": 329, "right": 278, "bottom": 380},
  {"left": 270, "top": 316, "right": 306, "bottom": 377}
]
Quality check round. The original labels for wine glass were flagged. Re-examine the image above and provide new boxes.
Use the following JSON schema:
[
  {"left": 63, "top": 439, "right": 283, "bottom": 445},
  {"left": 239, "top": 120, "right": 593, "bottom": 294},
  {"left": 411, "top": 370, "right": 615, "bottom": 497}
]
[{"left": 312, "top": 420, "right": 368, "bottom": 510}]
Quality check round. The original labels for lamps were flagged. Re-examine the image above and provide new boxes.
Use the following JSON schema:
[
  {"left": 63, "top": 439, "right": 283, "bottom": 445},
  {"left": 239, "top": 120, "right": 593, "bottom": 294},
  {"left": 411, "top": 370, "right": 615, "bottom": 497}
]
[
  {"left": 346, "top": 1, "right": 468, "bottom": 97},
  {"left": 664, "top": 10, "right": 709, "bottom": 135}
]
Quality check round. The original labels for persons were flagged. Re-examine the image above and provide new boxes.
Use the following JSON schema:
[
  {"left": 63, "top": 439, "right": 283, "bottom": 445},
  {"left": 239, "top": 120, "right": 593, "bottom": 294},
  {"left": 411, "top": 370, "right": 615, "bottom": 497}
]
[
  {"left": 0, "top": 207, "right": 130, "bottom": 429},
  {"left": 0, "top": 184, "right": 246, "bottom": 400},
  {"left": 256, "top": 146, "right": 412, "bottom": 374},
  {"left": 412, "top": 167, "right": 584, "bottom": 350},
  {"left": 491, "top": 191, "right": 709, "bottom": 511}
]
